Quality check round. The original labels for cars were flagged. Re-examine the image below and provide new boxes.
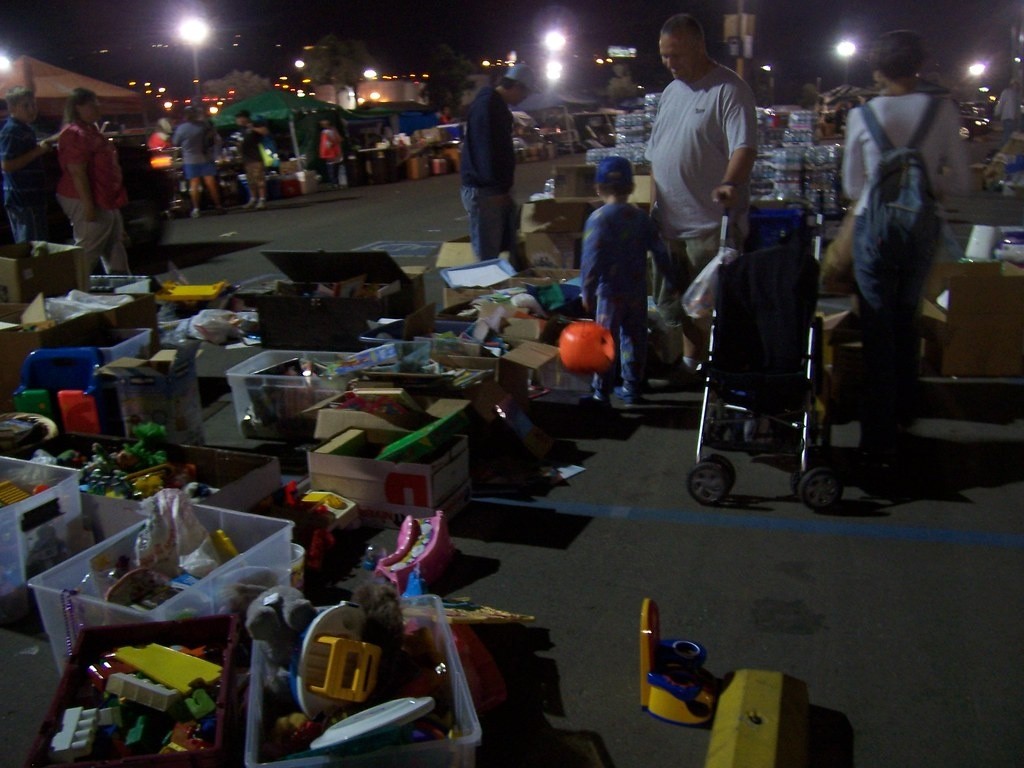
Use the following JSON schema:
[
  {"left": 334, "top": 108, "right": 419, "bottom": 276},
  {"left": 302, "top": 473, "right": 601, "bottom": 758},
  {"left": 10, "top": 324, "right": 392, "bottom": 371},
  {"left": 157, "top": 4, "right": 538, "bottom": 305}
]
[{"left": 0, "top": 133, "right": 169, "bottom": 273}]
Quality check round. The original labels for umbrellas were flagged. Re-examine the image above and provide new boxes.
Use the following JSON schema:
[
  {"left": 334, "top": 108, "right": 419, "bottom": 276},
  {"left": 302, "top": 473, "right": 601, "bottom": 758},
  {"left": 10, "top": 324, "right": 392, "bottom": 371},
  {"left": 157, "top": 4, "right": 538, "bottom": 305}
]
[
  {"left": 517, "top": 85, "right": 601, "bottom": 152},
  {"left": 348, "top": 101, "right": 432, "bottom": 117},
  {"left": 0, "top": 55, "right": 142, "bottom": 118},
  {"left": 204, "top": 87, "right": 354, "bottom": 172},
  {"left": 822, "top": 72, "right": 953, "bottom": 98}
]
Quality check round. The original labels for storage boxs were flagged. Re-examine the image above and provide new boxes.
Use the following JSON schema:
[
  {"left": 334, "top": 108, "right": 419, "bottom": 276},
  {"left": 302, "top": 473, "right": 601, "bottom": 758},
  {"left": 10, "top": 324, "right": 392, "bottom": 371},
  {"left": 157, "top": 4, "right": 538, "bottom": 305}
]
[{"left": 0, "top": 158, "right": 1024, "bottom": 768}]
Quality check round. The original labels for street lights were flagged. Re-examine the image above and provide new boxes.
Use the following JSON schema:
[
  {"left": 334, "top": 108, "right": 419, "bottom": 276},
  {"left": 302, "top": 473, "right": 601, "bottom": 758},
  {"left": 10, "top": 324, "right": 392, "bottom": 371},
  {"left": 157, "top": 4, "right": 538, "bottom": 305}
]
[
  {"left": 173, "top": 14, "right": 212, "bottom": 106},
  {"left": 834, "top": 38, "right": 858, "bottom": 82}
]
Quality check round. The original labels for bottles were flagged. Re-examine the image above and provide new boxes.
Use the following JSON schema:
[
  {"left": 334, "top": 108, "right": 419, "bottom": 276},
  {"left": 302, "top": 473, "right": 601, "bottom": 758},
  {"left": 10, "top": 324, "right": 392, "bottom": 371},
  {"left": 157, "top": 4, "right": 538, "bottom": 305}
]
[{"left": 583, "top": 89, "right": 849, "bottom": 219}]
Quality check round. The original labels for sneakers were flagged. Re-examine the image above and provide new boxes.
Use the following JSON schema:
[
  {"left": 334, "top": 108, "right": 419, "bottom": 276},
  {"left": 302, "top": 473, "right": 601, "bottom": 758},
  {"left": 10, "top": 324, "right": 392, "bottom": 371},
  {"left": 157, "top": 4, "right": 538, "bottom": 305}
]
[
  {"left": 645, "top": 370, "right": 703, "bottom": 394},
  {"left": 190, "top": 209, "right": 202, "bottom": 218},
  {"left": 593, "top": 391, "right": 607, "bottom": 402},
  {"left": 612, "top": 385, "right": 641, "bottom": 404},
  {"left": 216, "top": 207, "right": 227, "bottom": 214},
  {"left": 244, "top": 199, "right": 267, "bottom": 210}
]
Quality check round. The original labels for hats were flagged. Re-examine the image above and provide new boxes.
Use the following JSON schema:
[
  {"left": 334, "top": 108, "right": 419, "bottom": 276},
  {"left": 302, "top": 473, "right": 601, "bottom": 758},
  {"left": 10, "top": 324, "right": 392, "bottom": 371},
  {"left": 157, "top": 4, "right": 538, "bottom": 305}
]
[
  {"left": 505, "top": 64, "right": 536, "bottom": 92},
  {"left": 593, "top": 157, "right": 632, "bottom": 184}
]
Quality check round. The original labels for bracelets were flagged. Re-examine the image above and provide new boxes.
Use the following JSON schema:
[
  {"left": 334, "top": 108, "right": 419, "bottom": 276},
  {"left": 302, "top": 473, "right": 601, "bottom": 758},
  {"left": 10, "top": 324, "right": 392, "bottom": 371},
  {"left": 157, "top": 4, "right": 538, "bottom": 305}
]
[{"left": 719, "top": 180, "right": 742, "bottom": 194}]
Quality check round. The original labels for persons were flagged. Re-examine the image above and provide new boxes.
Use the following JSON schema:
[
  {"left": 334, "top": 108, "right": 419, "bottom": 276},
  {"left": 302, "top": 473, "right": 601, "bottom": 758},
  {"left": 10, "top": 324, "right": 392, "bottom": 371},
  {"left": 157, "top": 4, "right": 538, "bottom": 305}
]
[
  {"left": 582, "top": 12, "right": 757, "bottom": 408},
  {"left": 459, "top": 64, "right": 541, "bottom": 271},
  {"left": 147, "top": 106, "right": 269, "bottom": 218},
  {"left": 319, "top": 119, "right": 348, "bottom": 191},
  {"left": 842, "top": 28, "right": 956, "bottom": 460},
  {"left": 994, "top": 78, "right": 1021, "bottom": 145},
  {"left": 0, "top": 86, "right": 130, "bottom": 275},
  {"left": 440, "top": 106, "right": 455, "bottom": 125}
]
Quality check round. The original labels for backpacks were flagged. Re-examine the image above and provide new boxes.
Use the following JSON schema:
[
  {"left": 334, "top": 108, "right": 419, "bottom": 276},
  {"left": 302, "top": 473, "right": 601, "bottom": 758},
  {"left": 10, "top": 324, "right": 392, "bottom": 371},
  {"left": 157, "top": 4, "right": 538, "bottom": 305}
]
[{"left": 849, "top": 96, "right": 955, "bottom": 320}]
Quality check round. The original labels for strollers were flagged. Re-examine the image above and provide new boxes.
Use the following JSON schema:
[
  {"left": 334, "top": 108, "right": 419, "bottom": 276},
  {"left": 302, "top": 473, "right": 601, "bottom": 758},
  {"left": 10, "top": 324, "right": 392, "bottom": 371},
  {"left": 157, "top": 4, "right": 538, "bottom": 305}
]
[{"left": 687, "top": 193, "right": 844, "bottom": 511}]
